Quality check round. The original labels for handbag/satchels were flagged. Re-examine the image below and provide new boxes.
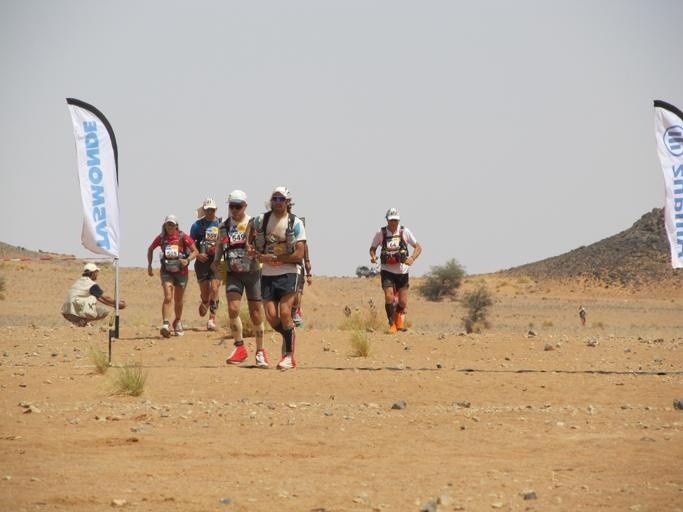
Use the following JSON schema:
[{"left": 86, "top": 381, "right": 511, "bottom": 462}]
[
  {"left": 162, "top": 258, "right": 182, "bottom": 273},
  {"left": 195, "top": 239, "right": 218, "bottom": 257},
  {"left": 222, "top": 246, "right": 258, "bottom": 273}
]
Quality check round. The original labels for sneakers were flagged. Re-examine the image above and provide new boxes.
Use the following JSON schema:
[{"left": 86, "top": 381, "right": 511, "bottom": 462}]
[
  {"left": 277, "top": 353, "right": 296, "bottom": 371},
  {"left": 171, "top": 319, "right": 183, "bottom": 337},
  {"left": 397, "top": 326, "right": 408, "bottom": 333},
  {"left": 255, "top": 348, "right": 270, "bottom": 368},
  {"left": 292, "top": 312, "right": 303, "bottom": 327},
  {"left": 159, "top": 324, "right": 171, "bottom": 338},
  {"left": 383, "top": 325, "right": 396, "bottom": 335},
  {"left": 224, "top": 344, "right": 248, "bottom": 365},
  {"left": 196, "top": 296, "right": 209, "bottom": 317},
  {"left": 206, "top": 319, "right": 216, "bottom": 330}
]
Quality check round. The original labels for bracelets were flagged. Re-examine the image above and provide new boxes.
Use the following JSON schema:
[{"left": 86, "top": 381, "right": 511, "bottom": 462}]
[
  {"left": 271, "top": 253, "right": 278, "bottom": 262},
  {"left": 410, "top": 255, "right": 415, "bottom": 261},
  {"left": 306, "top": 275, "right": 311, "bottom": 278},
  {"left": 185, "top": 258, "right": 190, "bottom": 263}
]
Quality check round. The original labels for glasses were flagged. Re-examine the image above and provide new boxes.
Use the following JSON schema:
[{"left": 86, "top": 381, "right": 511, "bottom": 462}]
[
  {"left": 229, "top": 204, "right": 242, "bottom": 210},
  {"left": 271, "top": 196, "right": 287, "bottom": 201}
]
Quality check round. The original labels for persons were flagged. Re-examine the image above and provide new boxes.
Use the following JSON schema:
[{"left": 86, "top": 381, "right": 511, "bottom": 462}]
[
  {"left": 59, "top": 262, "right": 128, "bottom": 324},
  {"left": 209, "top": 189, "right": 269, "bottom": 367},
  {"left": 189, "top": 198, "right": 223, "bottom": 331},
  {"left": 286, "top": 198, "right": 313, "bottom": 326},
  {"left": 146, "top": 214, "right": 199, "bottom": 337},
  {"left": 368, "top": 207, "right": 422, "bottom": 335},
  {"left": 243, "top": 186, "right": 306, "bottom": 369}
]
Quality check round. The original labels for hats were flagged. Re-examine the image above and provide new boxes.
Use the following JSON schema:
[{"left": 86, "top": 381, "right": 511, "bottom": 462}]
[
  {"left": 83, "top": 262, "right": 100, "bottom": 273},
  {"left": 202, "top": 197, "right": 218, "bottom": 211},
  {"left": 225, "top": 190, "right": 248, "bottom": 207},
  {"left": 386, "top": 207, "right": 401, "bottom": 221},
  {"left": 270, "top": 186, "right": 291, "bottom": 200},
  {"left": 162, "top": 214, "right": 177, "bottom": 225}
]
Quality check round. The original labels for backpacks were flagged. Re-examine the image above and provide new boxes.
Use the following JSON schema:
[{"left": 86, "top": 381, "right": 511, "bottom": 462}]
[{"left": 379, "top": 236, "right": 409, "bottom": 265}]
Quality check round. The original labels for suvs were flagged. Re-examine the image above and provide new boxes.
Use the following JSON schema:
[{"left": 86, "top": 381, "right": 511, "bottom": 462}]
[{"left": 353, "top": 265, "right": 376, "bottom": 279}]
